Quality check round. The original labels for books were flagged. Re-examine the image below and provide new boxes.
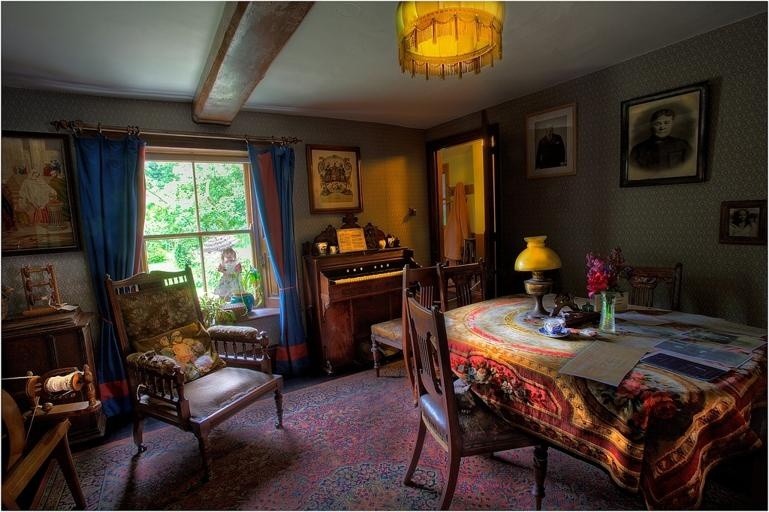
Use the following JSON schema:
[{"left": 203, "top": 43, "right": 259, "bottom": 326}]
[{"left": 336, "top": 228, "right": 367, "bottom": 253}]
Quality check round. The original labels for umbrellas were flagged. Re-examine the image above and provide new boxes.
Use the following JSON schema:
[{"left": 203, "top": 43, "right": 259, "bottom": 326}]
[{"left": 195, "top": 235, "right": 241, "bottom": 254}]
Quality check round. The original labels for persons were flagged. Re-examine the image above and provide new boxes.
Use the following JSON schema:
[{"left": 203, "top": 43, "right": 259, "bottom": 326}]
[
  {"left": 214, "top": 248, "right": 242, "bottom": 300},
  {"left": 10, "top": 157, "right": 70, "bottom": 224},
  {"left": 729, "top": 209, "right": 753, "bottom": 236},
  {"left": 628, "top": 108, "right": 693, "bottom": 171},
  {"left": 535, "top": 123, "right": 565, "bottom": 170}
]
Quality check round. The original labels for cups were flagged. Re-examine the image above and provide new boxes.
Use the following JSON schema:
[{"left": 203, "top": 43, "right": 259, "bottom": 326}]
[
  {"left": 315, "top": 242, "right": 340, "bottom": 256},
  {"left": 378, "top": 237, "right": 396, "bottom": 250},
  {"left": 544, "top": 316, "right": 566, "bottom": 336}
]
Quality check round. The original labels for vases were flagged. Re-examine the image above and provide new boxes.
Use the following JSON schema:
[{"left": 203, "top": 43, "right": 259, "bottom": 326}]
[
  {"left": 595, "top": 287, "right": 619, "bottom": 334},
  {"left": 229, "top": 291, "right": 256, "bottom": 314}
]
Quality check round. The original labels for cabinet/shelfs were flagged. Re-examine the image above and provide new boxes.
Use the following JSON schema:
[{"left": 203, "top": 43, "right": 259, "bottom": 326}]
[{"left": 2, "top": 307, "right": 108, "bottom": 450}]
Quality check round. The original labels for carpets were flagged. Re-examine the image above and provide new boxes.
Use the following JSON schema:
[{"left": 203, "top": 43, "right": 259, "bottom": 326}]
[{"left": 23, "top": 357, "right": 644, "bottom": 510}]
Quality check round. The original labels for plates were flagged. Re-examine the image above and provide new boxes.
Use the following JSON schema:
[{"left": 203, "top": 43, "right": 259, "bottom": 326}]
[{"left": 538, "top": 326, "right": 571, "bottom": 340}]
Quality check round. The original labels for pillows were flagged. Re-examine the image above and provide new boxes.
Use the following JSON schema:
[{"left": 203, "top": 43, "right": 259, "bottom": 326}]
[{"left": 127, "top": 316, "right": 227, "bottom": 392}]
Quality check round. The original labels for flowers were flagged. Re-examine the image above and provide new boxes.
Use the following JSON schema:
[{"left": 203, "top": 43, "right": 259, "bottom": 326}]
[
  {"left": 215, "top": 262, "right": 261, "bottom": 293},
  {"left": 580, "top": 246, "right": 629, "bottom": 320}
]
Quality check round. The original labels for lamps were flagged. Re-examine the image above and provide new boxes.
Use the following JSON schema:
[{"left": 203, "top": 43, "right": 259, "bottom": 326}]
[
  {"left": 391, "top": 1, "right": 507, "bottom": 85},
  {"left": 512, "top": 234, "right": 569, "bottom": 320}
]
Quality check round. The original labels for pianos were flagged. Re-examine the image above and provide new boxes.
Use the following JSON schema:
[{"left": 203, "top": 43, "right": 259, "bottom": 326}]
[{"left": 304, "top": 246, "right": 422, "bottom": 365}]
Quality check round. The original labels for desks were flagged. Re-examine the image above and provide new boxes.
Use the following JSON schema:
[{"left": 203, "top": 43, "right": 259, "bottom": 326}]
[{"left": 425, "top": 290, "right": 767, "bottom": 510}]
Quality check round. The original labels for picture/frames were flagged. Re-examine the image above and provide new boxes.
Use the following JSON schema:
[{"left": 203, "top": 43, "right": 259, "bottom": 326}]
[
  {"left": 718, "top": 199, "right": 767, "bottom": 248},
  {"left": 302, "top": 140, "right": 367, "bottom": 217},
  {"left": 2, "top": 127, "right": 84, "bottom": 254},
  {"left": 523, "top": 101, "right": 579, "bottom": 182},
  {"left": 619, "top": 80, "right": 711, "bottom": 189}
]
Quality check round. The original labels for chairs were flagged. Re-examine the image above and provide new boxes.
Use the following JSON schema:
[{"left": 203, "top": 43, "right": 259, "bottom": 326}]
[
  {"left": 101, "top": 262, "right": 286, "bottom": 483},
  {"left": 622, "top": 262, "right": 685, "bottom": 310},
  {"left": 369, "top": 260, "right": 453, "bottom": 378},
  {"left": 1, "top": 388, "right": 89, "bottom": 510},
  {"left": 401, "top": 286, "right": 551, "bottom": 510},
  {"left": 435, "top": 257, "right": 489, "bottom": 314}
]
